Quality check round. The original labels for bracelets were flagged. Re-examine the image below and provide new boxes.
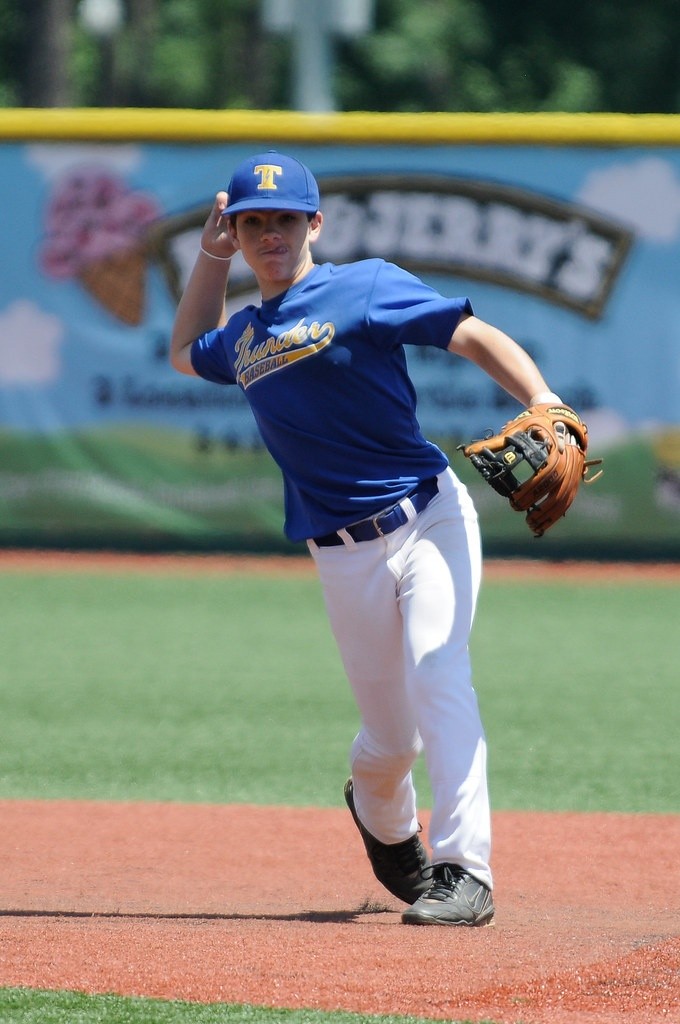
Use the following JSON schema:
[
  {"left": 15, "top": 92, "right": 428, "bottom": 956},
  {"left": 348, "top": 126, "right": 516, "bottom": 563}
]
[{"left": 199, "top": 243, "right": 235, "bottom": 262}]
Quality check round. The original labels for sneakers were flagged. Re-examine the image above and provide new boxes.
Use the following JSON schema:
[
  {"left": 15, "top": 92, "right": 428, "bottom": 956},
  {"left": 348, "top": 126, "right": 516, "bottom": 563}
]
[
  {"left": 401, "top": 862, "right": 495, "bottom": 927},
  {"left": 344, "top": 776, "right": 434, "bottom": 928}
]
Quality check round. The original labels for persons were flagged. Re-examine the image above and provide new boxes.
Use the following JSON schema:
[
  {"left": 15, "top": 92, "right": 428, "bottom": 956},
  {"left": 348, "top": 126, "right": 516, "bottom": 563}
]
[{"left": 170, "top": 151, "right": 584, "bottom": 926}]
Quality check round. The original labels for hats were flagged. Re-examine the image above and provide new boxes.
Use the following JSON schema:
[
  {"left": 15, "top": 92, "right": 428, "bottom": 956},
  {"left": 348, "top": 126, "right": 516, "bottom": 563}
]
[{"left": 220, "top": 150, "right": 320, "bottom": 215}]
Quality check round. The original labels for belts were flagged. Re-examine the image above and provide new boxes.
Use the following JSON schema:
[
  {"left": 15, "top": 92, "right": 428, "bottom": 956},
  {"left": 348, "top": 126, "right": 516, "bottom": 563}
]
[{"left": 312, "top": 477, "right": 439, "bottom": 547}]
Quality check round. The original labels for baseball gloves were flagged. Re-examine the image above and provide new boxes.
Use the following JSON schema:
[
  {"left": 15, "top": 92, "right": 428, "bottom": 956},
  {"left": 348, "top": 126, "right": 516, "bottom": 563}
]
[{"left": 460, "top": 403, "right": 589, "bottom": 538}]
